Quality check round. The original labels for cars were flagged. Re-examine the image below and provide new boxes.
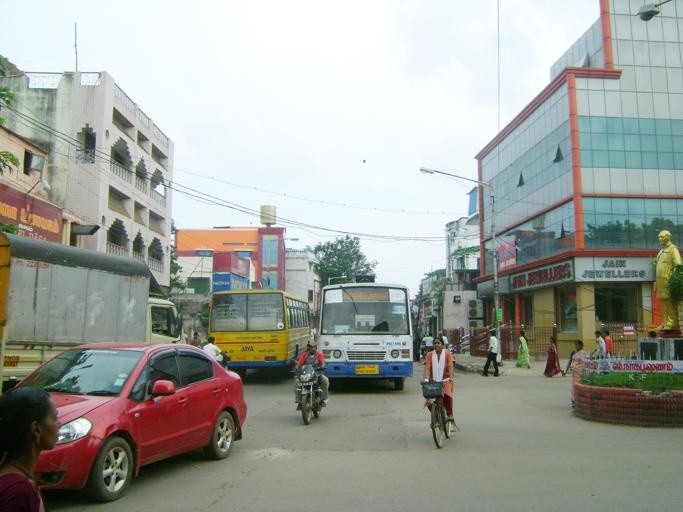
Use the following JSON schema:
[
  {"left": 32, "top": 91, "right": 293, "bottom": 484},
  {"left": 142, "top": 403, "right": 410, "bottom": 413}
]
[{"left": 1, "top": 341, "right": 247, "bottom": 500}]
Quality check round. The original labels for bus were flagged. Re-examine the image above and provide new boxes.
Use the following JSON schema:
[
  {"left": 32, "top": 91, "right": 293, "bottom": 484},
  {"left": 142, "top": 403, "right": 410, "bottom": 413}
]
[
  {"left": 209, "top": 289, "right": 313, "bottom": 374},
  {"left": 317, "top": 282, "right": 414, "bottom": 389}
]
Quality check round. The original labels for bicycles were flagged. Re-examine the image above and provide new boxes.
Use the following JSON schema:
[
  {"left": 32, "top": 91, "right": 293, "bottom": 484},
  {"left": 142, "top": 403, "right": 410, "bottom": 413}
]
[{"left": 422, "top": 376, "right": 454, "bottom": 447}]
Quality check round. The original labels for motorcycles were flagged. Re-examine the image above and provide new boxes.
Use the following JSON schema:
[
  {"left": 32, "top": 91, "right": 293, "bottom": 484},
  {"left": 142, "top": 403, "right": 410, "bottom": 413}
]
[{"left": 292, "top": 361, "right": 328, "bottom": 425}]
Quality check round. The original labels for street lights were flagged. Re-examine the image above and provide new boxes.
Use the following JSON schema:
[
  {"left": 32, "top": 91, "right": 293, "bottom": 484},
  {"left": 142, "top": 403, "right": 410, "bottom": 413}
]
[{"left": 422, "top": 167, "right": 501, "bottom": 365}]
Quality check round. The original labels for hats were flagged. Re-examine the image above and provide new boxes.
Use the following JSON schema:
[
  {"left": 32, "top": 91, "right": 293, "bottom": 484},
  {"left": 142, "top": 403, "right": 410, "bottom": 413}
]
[{"left": 307, "top": 340, "right": 317, "bottom": 347}]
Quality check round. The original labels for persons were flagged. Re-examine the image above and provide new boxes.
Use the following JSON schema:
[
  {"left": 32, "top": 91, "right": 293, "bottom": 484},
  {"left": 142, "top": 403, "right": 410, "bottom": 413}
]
[
  {"left": 589, "top": 330, "right": 608, "bottom": 360},
  {"left": 190, "top": 330, "right": 200, "bottom": 347},
  {"left": 292, "top": 339, "right": 330, "bottom": 411},
  {"left": 603, "top": 328, "right": 613, "bottom": 357},
  {"left": 563, "top": 339, "right": 587, "bottom": 408},
  {"left": 413, "top": 329, "right": 448, "bottom": 364},
  {"left": 652, "top": 230, "right": 682, "bottom": 332},
  {"left": 201, "top": 336, "right": 223, "bottom": 362},
  {"left": 513, "top": 330, "right": 532, "bottom": 369},
  {"left": 543, "top": 336, "right": 565, "bottom": 377},
  {"left": 0, "top": 384, "right": 67, "bottom": 512},
  {"left": 480, "top": 330, "right": 500, "bottom": 377},
  {"left": 421, "top": 336, "right": 460, "bottom": 433}
]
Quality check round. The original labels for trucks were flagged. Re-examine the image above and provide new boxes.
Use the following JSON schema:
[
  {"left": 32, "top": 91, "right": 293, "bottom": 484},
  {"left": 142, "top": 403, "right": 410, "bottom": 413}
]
[{"left": 0, "top": 231, "right": 186, "bottom": 386}]
